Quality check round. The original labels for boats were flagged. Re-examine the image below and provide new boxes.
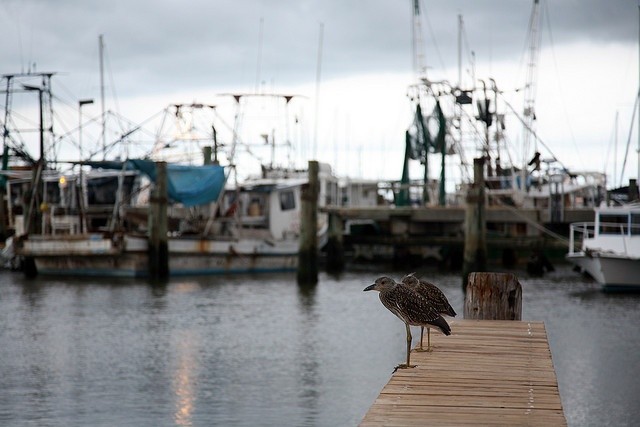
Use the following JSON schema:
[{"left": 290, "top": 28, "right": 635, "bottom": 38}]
[
  {"left": 15, "top": 160, "right": 329, "bottom": 275},
  {"left": 568, "top": 207, "right": 640, "bottom": 287}
]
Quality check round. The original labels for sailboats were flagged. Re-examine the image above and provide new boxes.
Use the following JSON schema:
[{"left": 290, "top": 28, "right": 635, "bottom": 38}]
[
  {"left": 3, "top": 33, "right": 142, "bottom": 231},
  {"left": 455, "top": 0, "right": 607, "bottom": 223},
  {"left": 218, "top": 17, "right": 346, "bottom": 237},
  {"left": 566, "top": 91, "right": 639, "bottom": 233},
  {"left": 317, "top": 1, "right": 541, "bottom": 267},
  {"left": 0, "top": 67, "right": 53, "bottom": 269}
]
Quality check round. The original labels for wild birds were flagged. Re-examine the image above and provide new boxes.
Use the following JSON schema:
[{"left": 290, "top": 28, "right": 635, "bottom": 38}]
[
  {"left": 400, "top": 271, "right": 457, "bottom": 353},
  {"left": 363, "top": 276, "right": 452, "bottom": 374}
]
